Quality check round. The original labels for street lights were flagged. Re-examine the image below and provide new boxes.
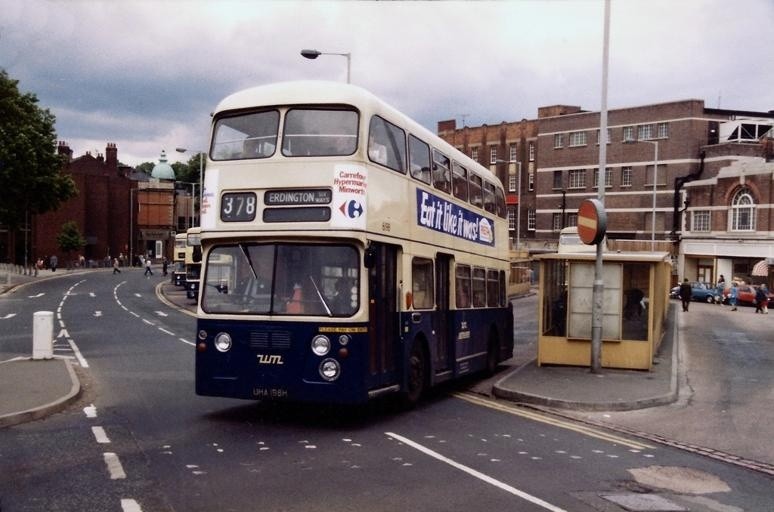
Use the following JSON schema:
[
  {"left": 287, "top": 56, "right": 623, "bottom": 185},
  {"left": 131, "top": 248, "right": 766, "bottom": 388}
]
[
  {"left": 175, "top": 180, "right": 196, "bottom": 228},
  {"left": 300, "top": 50, "right": 351, "bottom": 84},
  {"left": 129, "top": 187, "right": 146, "bottom": 254},
  {"left": 175, "top": 147, "right": 204, "bottom": 210},
  {"left": 621, "top": 138, "right": 658, "bottom": 252}
]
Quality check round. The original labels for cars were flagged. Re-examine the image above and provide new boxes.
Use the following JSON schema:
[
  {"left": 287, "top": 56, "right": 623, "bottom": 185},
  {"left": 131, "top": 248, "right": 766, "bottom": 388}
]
[{"left": 669, "top": 277, "right": 773, "bottom": 305}]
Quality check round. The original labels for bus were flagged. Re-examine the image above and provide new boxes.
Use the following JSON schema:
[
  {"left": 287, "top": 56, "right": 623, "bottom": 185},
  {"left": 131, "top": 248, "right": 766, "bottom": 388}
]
[
  {"left": 171, "top": 227, "right": 233, "bottom": 302},
  {"left": 196, "top": 81, "right": 514, "bottom": 410}
]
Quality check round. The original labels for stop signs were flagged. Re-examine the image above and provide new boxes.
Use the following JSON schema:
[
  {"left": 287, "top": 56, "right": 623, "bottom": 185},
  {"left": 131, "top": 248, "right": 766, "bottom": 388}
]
[{"left": 576, "top": 196, "right": 606, "bottom": 246}]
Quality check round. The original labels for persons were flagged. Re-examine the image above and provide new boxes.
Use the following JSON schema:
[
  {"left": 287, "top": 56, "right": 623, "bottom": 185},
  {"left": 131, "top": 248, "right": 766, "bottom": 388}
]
[
  {"left": 229, "top": 263, "right": 272, "bottom": 310},
  {"left": 34, "top": 252, "right": 168, "bottom": 276},
  {"left": 717, "top": 275, "right": 770, "bottom": 313},
  {"left": 522, "top": 268, "right": 534, "bottom": 284},
  {"left": 368, "top": 130, "right": 388, "bottom": 165},
  {"left": 322, "top": 127, "right": 355, "bottom": 156},
  {"left": 680, "top": 278, "right": 692, "bottom": 311},
  {"left": 231, "top": 136, "right": 266, "bottom": 159}
]
